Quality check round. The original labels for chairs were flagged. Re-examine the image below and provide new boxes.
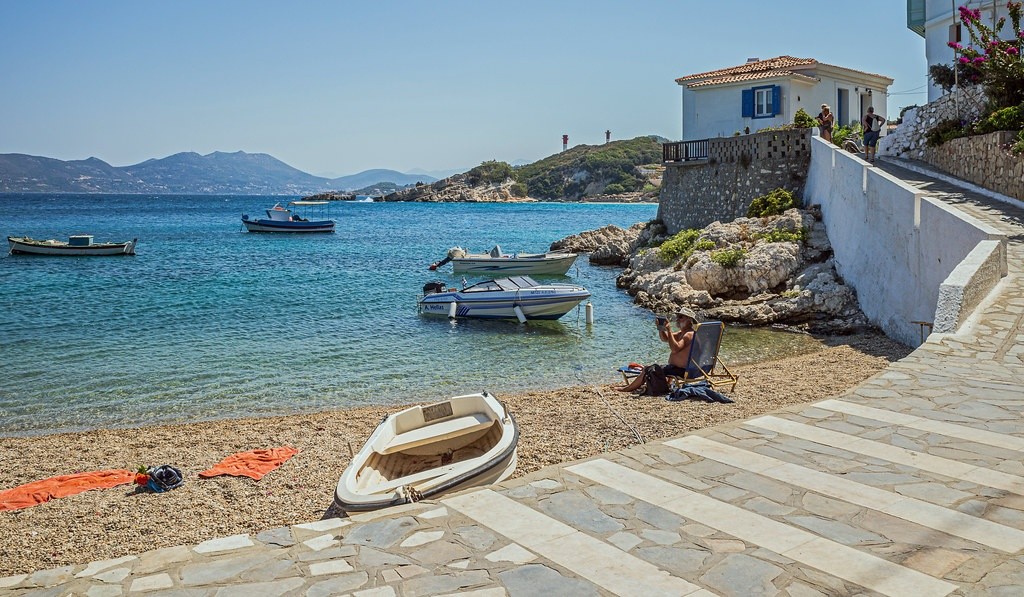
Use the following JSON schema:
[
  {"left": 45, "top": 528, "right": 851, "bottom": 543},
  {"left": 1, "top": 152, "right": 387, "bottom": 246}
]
[{"left": 617, "top": 322, "right": 740, "bottom": 394}]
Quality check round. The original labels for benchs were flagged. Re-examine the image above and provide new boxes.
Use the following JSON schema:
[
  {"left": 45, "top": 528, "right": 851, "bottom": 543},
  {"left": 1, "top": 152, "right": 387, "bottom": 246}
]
[
  {"left": 385, "top": 412, "right": 493, "bottom": 457},
  {"left": 359, "top": 456, "right": 479, "bottom": 494}
]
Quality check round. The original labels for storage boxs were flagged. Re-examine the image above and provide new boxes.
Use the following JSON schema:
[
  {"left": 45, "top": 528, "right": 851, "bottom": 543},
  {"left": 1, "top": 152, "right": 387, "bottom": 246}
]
[{"left": 69, "top": 235, "right": 94, "bottom": 246}]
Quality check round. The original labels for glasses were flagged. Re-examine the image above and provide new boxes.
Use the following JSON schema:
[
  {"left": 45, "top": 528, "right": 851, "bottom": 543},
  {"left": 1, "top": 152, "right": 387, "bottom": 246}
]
[{"left": 676, "top": 314, "right": 683, "bottom": 318}]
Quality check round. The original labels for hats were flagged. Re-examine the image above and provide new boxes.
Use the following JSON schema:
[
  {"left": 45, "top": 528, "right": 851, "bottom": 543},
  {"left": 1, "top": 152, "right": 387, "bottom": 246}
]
[{"left": 675, "top": 307, "right": 699, "bottom": 324}]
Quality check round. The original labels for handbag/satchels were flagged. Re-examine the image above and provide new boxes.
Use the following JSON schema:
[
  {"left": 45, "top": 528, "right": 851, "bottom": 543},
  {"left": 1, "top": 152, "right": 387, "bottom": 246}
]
[{"left": 644, "top": 365, "right": 669, "bottom": 396}]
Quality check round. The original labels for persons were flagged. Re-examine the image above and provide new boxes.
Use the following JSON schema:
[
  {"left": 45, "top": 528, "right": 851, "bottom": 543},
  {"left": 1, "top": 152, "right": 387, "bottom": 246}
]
[
  {"left": 616, "top": 307, "right": 699, "bottom": 393},
  {"left": 862, "top": 106, "right": 886, "bottom": 162},
  {"left": 815, "top": 104, "right": 834, "bottom": 144}
]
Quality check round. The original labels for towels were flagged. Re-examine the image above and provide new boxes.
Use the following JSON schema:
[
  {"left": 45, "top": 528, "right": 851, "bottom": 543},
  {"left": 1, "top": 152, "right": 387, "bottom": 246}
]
[
  {"left": 664, "top": 380, "right": 734, "bottom": 404},
  {"left": 197, "top": 444, "right": 299, "bottom": 481},
  {"left": 0, "top": 469, "right": 139, "bottom": 511}
]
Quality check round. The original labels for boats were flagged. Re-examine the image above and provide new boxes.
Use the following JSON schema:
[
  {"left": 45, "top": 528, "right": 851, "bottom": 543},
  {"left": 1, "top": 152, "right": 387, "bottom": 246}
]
[
  {"left": 241, "top": 202, "right": 336, "bottom": 235},
  {"left": 334, "top": 390, "right": 522, "bottom": 518},
  {"left": 417, "top": 275, "right": 590, "bottom": 323},
  {"left": 428, "top": 244, "right": 581, "bottom": 277},
  {"left": 8, "top": 235, "right": 138, "bottom": 258}
]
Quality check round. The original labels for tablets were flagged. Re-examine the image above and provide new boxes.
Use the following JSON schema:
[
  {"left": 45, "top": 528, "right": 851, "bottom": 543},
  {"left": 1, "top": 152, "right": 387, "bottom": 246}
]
[{"left": 657, "top": 317, "right": 669, "bottom": 331}]
